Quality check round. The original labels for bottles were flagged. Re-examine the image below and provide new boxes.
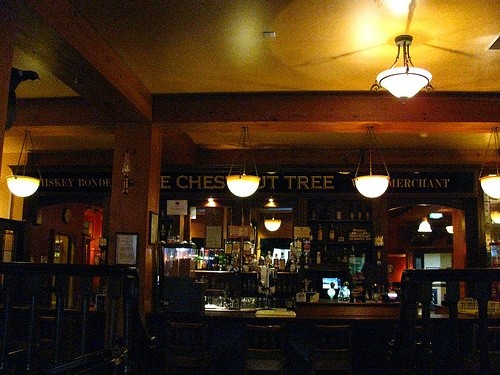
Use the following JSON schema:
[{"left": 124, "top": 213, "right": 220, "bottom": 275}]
[
  {"left": 311, "top": 223, "right": 401, "bottom": 303},
  {"left": 196, "top": 237, "right": 311, "bottom": 271},
  {"left": 310, "top": 200, "right": 371, "bottom": 220},
  {"left": 195, "top": 275, "right": 313, "bottom": 311}
]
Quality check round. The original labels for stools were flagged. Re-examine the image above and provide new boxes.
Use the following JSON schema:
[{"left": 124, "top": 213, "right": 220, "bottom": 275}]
[
  {"left": 309, "top": 323, "right": 354, "bottom": 375},
  {"left": 385, "top": 326, "right": 434, "bottom": 375},
  {"left": 167, "top": 322, "right": 210, "bottom": 375},
  {"left": 464, "top": 323, "right": 500, "bottom": 375},
  {"left": 243, "top": 323, "right": 287, "bottom": 375}
]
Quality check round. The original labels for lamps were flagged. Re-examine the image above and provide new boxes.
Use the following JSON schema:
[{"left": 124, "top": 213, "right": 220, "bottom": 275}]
[
  {"left": 478, "top": 127, "right": 500, "bottom": 198},
  {"left": 122, "top": 172, "right": 134, "bottom": 194},
  {"left": 350, "top": 125, "right": 390, "bottom": 198},
  {"left": 6, "top": 130, "right": 42, "bottom": 198},
  {"left": 226, "top": 126, "right": 261, "bottom": 197},
  {"left": 264, "top": 214, "right": 282, "bottom": 232},
  {"left": 418, "top": 217, "right": 432, "bottom": 233},
  {"left": 371, "top": 35, "right": 436, "bottom": 105}
]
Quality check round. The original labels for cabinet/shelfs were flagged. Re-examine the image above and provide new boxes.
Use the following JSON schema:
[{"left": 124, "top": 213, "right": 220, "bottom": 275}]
[{"left": 306, "top": 198, "right": 373, "bottom": 272}]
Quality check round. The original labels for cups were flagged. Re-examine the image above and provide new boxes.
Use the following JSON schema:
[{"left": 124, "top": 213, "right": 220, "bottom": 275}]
[
  {"left": 167, "top": 235, "right": 180, "bottom": 244},
  {"left": 41, "top": 256, "right": 48, "bottom": 263}
]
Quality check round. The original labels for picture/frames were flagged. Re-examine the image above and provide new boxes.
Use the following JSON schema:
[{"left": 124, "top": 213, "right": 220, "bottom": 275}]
[
  {"left": 149, "top": 211, "right": 159, "bottom": 245},
  {"left": 115, "top": 232, "right": 138, "bottom": 265}
]
